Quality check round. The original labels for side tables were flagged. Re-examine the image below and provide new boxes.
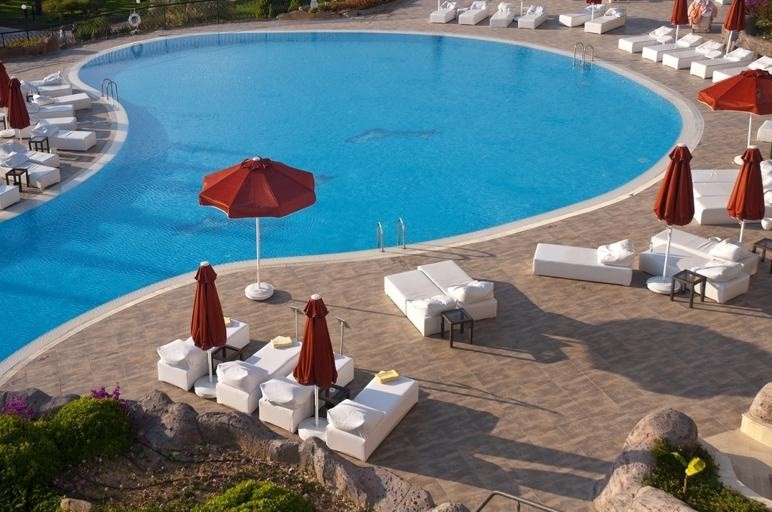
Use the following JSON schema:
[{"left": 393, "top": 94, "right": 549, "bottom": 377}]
[{"left": 752, "top": 238, "right": 772, "bottom": 275}]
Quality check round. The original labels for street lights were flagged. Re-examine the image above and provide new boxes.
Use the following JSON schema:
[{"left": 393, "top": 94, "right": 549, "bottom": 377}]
[
  {"left": 135, "top": 0, "right": 141, "bottom": 11},
  {"left": 21, "top": 4, "right": 30, "bottom": 40}
]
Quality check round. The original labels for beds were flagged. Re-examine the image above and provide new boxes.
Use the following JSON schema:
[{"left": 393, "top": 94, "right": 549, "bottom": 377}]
[
  {"left": 385, "top": 261, "right": 500, "bottom": 340},
  {"left": 618, "top": 26, "right": 772, "bottom": 85},
  {"left": 428, "top": 1, "right": 547, "bottom": 30},
  {"left": 0, "top": 78, "right": 97, "bottom": 212},
  {"left": 690, "top": 159, "right": 772, "bottom": 226},
  {"left": 157, "top": 321, "right": 420, "bottom": 462},
  {"left": 558, "top": 0, "right": 603, "bottom": 28},
  {"left": 638, "top": 227, "right": 759, "bottom": 304},
  {"left": 533, "top": 241, "right": 633, "bottom": 286},
  {"left": 583, "top": 8, "right": 624, "bottom": 34}
]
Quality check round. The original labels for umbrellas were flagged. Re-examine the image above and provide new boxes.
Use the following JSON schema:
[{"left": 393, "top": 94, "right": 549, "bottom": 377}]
[
  {"left": 293, "top": 293, "right": 339, "bottom": 426},
  {"left": 696, "top": 68, "right": 771, "bottom": 147},
  {"left": 0, "top": 63, "right": 11, "bottom": 130},
  {"left": 726, "top": 144, "right": 764, "bottom": 245},
  {"left": 190, "top": 262, "right": 228, "bottom": 383},
  {"left": 648, "top": 141, "right": 694, "bottom": 294},
  {"left": 6, "top": 78, "right": 30, "bottom": 144},
  {"left": 721, "top": 0, "right": 745, "bottom": 54},
  {"left": 198, "top": 155, "right": 317, "bottom": 289},
  {"left": 670, "top": 0, "right": 690, "bottom": 47},
  {"left": 585, "top": 1, "right": 600, "bottom": 20}
]
